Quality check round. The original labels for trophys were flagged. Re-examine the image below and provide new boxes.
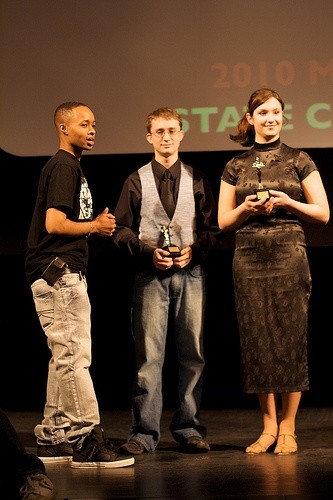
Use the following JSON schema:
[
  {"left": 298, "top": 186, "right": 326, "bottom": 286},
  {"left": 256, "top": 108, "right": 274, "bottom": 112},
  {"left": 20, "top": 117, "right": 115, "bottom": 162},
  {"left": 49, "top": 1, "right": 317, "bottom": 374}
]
[
  {"left": 160, "top": 225, "right": 181, "bottom": 257},
  {"left": 252, "top": 157, "right": 270, "bottom": 201}
]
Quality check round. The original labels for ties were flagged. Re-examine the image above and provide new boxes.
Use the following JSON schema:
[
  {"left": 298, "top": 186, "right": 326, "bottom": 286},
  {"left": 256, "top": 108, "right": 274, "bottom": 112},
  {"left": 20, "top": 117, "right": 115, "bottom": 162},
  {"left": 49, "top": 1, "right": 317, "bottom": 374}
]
[{"left": 161, "top": 169, "right": 177, "bottom": 221}]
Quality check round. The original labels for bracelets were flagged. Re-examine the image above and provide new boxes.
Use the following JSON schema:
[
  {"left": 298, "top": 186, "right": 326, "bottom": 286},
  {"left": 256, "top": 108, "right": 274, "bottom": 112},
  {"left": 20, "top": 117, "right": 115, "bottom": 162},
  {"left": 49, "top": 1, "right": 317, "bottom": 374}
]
[{"left": 86, "top": 222, "right": 92, "bottom": 238}]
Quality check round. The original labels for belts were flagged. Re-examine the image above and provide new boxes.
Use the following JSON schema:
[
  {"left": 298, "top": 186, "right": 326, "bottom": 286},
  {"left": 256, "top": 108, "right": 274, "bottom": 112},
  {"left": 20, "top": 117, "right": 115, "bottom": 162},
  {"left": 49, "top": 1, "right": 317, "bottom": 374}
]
[{"left": 64, "top": 268, "right": 79, "bottom": 274}]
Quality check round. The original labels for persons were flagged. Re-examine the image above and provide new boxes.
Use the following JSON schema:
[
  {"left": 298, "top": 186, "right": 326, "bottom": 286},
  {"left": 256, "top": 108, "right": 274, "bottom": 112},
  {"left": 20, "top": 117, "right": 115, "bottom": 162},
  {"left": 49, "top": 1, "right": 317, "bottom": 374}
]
[
  {"left": 25, "top": 102, "right": 135, "bottom": 468},
  {"left": 112, "top": 107, "right": 214, "bottom": 454},
  {"left": 218, "top": 89, "right": 330, "bottom": 455}
]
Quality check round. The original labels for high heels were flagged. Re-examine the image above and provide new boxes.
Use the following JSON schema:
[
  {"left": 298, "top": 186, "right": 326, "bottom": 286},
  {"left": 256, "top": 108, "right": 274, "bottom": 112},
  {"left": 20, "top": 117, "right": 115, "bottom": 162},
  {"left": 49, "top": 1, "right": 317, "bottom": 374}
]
[
  {"left": 275, "top": 433, "right": 298, "bottom": 455},
  {"left": 245, "top": 433, "right": 278, "bottom": 455}
]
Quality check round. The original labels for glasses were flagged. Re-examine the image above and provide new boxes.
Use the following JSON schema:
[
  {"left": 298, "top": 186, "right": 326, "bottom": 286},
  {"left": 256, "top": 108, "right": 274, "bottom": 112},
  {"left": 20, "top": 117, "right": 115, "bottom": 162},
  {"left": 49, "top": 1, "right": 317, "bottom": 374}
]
[{"left": 151, "top": 128, "right": 183, "bottom": 138}]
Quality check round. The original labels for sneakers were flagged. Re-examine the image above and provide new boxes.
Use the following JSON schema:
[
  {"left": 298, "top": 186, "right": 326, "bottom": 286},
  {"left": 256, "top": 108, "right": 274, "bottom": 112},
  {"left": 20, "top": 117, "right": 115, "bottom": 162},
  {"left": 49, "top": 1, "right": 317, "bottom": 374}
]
[
  {"left": 120, "top": 439, "right": 146, "bottom": 454},
  {"left": 180, "top": 436, "right": 211, "bottom": 452},
  {"left": 37, "top": 441, "right": 74, "bottom": 463},
  {"left": 19, "top": 472, "right": 57, "bottom": 500},
  {"left": 72, "top": 427, "right": 135, "bottom": 468}
]
